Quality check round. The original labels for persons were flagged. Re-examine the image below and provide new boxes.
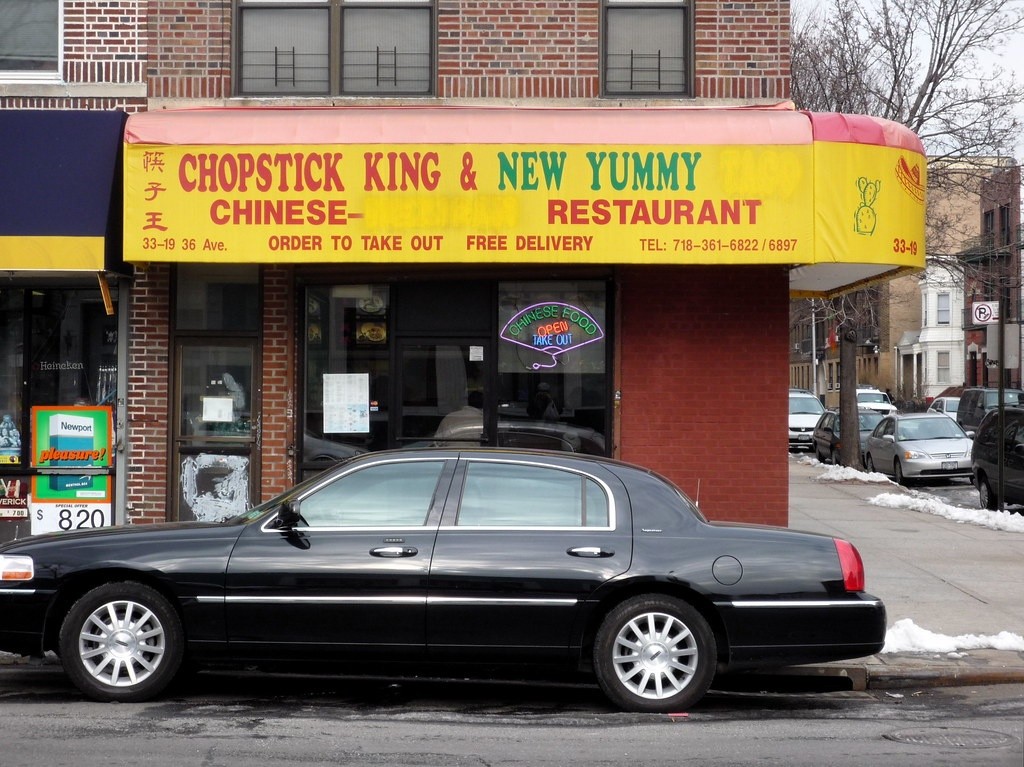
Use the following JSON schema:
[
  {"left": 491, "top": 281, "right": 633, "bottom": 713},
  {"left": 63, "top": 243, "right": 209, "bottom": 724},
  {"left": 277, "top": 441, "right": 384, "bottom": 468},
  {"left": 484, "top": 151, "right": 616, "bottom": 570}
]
[
  {"left": 883, "top": 388, "right": 895, "bottom": 403},
  {"left": 526, "top": 382, "right": 559, "bottom": 418},
  {"left": 886, "top": 423, "right": 893, "bottom": 435}
]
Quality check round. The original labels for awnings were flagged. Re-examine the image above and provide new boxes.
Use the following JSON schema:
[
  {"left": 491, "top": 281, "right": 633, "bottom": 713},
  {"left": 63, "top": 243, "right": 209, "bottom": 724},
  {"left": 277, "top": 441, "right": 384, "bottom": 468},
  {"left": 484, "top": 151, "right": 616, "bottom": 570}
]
[
  {"left": 1, "top": 108, "right": 126, "bottom": 273},
  {"left": 122, "top": 102, "right": 927, "bottom": 299}
]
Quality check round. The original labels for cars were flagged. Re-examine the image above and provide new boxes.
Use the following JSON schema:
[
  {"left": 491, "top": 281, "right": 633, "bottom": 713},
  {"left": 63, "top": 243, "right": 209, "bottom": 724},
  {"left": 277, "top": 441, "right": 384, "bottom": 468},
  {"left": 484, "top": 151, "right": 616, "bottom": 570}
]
[
  {"left": 812, "top": 408, "right": 890, "bottom": 466},
  {"left": 864, "top": 413, "right": 978, "bottom": 486},
  {"left": 968, "top": 406, "right": 1024, "bottom": 514},
  {"left": 926, "top": 395, "right": 961, "bottom": 423},
  {"left": 178, "top": 412, "right": 368, "bottom": 475},
  {"left": 432, "top": 424, "right": 607, "bottom": 456},
  {"left": 0, "top": 443, "right": 892, "bottom": 714}
]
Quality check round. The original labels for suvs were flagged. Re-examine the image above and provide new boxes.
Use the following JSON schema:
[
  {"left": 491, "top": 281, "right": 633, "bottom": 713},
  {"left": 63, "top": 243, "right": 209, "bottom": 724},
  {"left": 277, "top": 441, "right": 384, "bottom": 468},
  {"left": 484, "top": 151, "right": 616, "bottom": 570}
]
[
  {"left": 856, "top": 388, "right": 899, "bottom": 418},
  {"left": 789, "top": 388, "right": 828, "bottom": 450},
  {"left": 957, "top": 387, "right": 1024, "bottom": 438}
]
